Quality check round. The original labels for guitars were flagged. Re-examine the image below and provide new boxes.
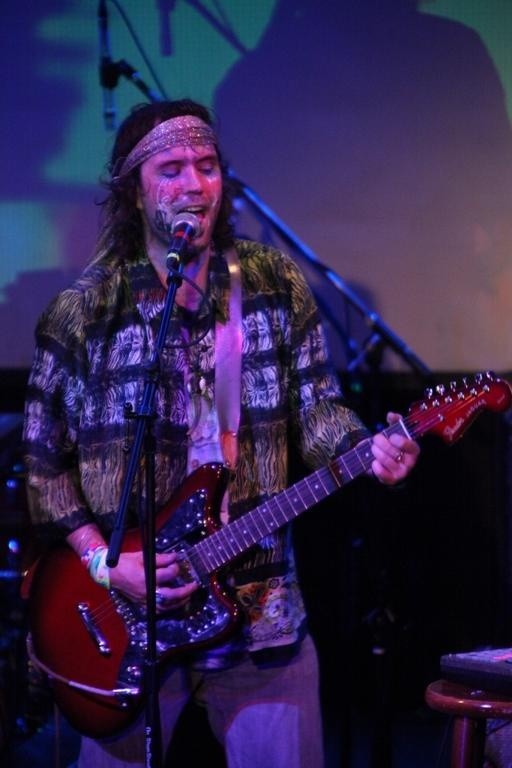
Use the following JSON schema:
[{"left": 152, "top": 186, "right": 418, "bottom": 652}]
[{"left": 21, "top": 372, "right": 512, "bottom": 737}]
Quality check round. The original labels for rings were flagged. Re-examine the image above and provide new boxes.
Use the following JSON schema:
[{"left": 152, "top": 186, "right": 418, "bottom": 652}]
[
  {"left": 394, "top": 451, "right": 404, "bottom": 463},
  {"left": 155, "top": 586, "right": 169, "bottom": 605}
]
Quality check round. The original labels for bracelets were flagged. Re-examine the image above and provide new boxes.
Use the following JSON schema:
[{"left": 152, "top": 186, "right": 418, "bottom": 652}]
[{"left": 80, "top": 543, "right": 110, "bottom": 591}]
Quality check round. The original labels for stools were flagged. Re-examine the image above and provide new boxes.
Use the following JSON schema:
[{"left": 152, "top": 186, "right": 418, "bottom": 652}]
[{"left": 425, "top": 647, "right": 511, "bottom": 768}]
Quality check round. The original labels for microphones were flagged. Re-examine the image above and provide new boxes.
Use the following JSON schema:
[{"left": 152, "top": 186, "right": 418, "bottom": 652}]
[{"left": 164, "top": 212, "right": 201, "bottom": 271}]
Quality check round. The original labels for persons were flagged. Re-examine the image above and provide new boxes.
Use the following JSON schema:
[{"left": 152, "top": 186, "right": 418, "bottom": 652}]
[{"left": 24, "top": 96, "right": 421, "bottom": 768}]
